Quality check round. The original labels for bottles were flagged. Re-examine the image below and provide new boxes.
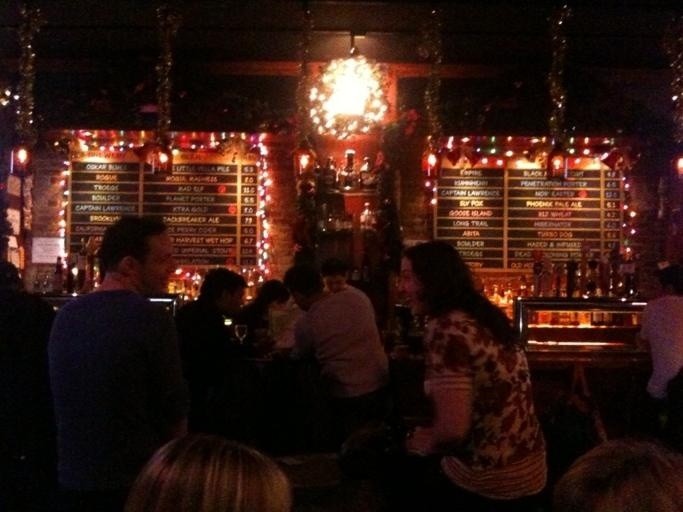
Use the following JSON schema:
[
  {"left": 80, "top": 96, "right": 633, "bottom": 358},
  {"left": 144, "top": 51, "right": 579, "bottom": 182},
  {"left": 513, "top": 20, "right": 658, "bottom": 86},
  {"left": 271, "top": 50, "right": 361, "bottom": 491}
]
[
  {"left": 314, "top": 150, "right": 377, "bottom": 193},
  {"left": 360, "top": 202, "right": 374, "bottom": 231},
  {"left": 483, "top": 252, "right": 634, "bottom": 326},
  {"left": 167, "top": 266, "right": 266, "bottom": 302},
  {"left": 31, "top": 256, "right": 94, "bottom": 294}
]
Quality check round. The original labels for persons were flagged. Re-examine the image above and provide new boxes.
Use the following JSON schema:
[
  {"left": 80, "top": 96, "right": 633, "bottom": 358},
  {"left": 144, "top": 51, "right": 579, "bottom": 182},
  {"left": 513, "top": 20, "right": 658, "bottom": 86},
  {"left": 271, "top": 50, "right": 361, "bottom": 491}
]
[{"left": 1, "top": 212, "right": 683, "bottom": 512}]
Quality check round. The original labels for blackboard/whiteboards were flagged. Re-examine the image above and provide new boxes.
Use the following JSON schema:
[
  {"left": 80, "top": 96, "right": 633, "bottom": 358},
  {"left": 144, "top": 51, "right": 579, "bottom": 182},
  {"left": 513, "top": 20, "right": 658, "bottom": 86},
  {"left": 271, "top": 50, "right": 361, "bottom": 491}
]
[
  {"left": 433, "top": 166, "right": 624, "bottom": 275},
  {"left": 67, "top": 157, "right": 261, "bottom": 271}
]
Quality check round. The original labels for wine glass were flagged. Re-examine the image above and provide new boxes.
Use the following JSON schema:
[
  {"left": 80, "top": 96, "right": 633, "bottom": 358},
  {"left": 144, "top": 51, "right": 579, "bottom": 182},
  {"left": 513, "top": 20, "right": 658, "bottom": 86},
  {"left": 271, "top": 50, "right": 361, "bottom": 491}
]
[{"left": 234, "top": 325, "right": 248, "bottom": 344}]
